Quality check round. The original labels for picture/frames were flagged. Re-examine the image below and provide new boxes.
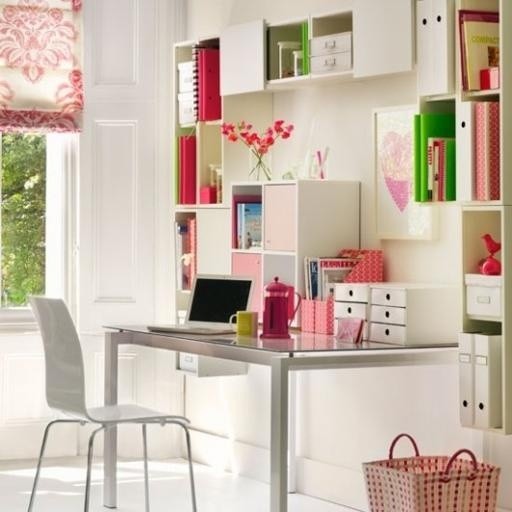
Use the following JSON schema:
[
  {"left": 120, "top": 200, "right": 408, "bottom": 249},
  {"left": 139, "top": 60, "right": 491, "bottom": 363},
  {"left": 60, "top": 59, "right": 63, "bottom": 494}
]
[{"left": 370, "top": 103, "right": 441, "bottom": 241}]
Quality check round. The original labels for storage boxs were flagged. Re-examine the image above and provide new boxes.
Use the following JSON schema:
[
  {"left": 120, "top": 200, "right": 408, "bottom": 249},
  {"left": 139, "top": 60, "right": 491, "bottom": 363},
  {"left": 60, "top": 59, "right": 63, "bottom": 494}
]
[{"left": 463, "top": 273, "right": 502, "bottom": 320}]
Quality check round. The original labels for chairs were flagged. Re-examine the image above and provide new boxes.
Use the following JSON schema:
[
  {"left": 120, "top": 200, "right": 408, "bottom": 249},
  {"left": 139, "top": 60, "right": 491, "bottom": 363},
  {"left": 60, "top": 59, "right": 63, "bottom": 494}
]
[{"left": 23, "top": 293, "right": 197, "bottom": 512}]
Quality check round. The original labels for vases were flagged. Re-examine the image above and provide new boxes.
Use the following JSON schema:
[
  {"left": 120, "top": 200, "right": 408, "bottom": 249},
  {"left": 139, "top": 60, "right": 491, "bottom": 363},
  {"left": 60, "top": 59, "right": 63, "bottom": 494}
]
[{"left": 248, "top": 150, "right": 273, "bottom": 183}]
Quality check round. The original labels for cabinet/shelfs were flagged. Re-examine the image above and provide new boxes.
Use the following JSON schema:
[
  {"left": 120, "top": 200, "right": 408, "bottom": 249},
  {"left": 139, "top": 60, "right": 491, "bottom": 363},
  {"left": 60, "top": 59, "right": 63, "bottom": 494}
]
[
  {"left": 170, "top": 37, "right": 274, "bottom": 325},
  {"left": 452, "top": 0, "right": 512, "bottom": 436},
  {"left": 218, "top": 0, "right": 417, "bottom": 98},
  {"left": 229, "top": 179, "right": 361, "bottom": 329}
]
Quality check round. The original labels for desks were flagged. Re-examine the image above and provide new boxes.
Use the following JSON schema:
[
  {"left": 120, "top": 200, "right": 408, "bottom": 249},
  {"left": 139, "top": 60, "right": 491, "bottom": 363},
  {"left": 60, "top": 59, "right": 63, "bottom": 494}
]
[{"left": 99, "top": 320, "right": 460, "bottom": 512}]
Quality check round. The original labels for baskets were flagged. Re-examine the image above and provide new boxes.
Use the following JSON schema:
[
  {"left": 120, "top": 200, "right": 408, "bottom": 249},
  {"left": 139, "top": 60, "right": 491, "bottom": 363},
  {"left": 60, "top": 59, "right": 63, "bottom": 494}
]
[{"left": 362, "top": 433, "right": 503, "bottom": 512}]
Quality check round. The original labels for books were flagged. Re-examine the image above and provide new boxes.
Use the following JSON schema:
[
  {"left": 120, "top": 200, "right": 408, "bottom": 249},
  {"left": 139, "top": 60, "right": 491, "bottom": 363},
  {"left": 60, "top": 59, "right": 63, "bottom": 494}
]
[
  {"left": 170, "top": 38, "right": 227, "bottom": 298},
  {"left": 303, "top": 252, "right": 374, "bottom": 336},
  {"left": 231, "top": 193, "right": 262, "bottom": 253},
  {"left": 411, "top": 7, "right": 503, "bottom": 204}
]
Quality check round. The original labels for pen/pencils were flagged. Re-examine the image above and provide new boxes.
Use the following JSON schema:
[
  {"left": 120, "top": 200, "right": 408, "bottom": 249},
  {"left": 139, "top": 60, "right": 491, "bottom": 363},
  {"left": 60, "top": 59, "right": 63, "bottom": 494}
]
[{"left": 317, "top": 151, "right": 324, "bottom": 179}]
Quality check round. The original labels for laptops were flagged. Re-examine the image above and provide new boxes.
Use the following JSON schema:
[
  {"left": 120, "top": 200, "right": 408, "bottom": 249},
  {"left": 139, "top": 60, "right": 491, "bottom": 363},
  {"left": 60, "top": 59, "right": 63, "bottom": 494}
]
[{"left": 147, "top": 273, "right": 255, "bottom": 335}]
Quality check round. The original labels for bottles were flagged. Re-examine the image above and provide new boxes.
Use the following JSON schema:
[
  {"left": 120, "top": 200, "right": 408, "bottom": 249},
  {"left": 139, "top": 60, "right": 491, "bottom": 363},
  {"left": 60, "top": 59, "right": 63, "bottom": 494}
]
[{"left": 259, "top": 276, "right": 303, "bottom": 340}]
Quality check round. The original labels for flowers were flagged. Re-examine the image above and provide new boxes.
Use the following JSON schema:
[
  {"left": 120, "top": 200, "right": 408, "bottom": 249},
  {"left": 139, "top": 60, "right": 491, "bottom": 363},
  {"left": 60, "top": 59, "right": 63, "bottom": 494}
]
[{"left": 218, "top": 117, "right": 294, "bottom": 181}]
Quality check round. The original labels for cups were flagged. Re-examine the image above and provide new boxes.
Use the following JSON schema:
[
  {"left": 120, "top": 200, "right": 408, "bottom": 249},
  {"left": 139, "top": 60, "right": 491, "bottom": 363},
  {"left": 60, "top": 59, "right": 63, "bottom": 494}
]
[{"left": 228, "top": 310, "right": 259, "bottom": 339}]
